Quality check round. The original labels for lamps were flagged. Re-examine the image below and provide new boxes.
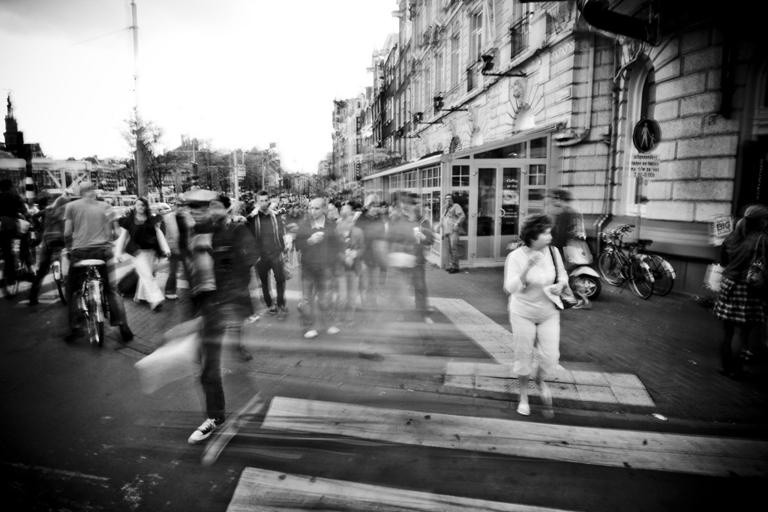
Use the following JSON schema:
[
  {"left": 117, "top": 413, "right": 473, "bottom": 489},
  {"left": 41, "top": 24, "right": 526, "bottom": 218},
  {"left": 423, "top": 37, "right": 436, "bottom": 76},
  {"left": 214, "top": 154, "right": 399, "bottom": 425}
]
[
  {"left": 432, "top": 94, "right": 468, "bottom": 112},
  {"left": 479, "top": 51, "right": 527, "bottom": 80},
  {"left": 413, "top": 111, "right": 443, "bottom": 124},
  {"left": 395, "top": 127, "right": 421, "bottom": 138}
]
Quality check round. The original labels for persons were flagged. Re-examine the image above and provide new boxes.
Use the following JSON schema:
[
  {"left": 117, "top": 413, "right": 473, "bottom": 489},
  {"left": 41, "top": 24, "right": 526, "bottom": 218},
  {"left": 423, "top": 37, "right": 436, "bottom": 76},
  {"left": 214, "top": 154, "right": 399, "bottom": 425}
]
[
  {"left": 711, "top": 204, "right": 766, "bottom": 377},
  {"left": 438, "top": 192, "right": 465, "bottom": 276},
  {"left": 547, "top": 183, "right": 587, "bottom": 247},
  {"left": 638, "top": 122, "right": 649, "bottom": 148},
  {"left": 648, "top": 131, "right": 655, "bottom": 149},
  {"left": 501, "top": 211, "right": 569, "bottom": 418}
]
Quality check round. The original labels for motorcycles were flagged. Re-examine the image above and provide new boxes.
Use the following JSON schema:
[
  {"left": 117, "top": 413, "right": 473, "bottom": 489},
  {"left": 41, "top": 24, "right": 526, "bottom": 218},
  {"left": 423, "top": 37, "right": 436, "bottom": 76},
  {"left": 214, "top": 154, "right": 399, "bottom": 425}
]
[{"left": 563, "top": 238, "right": 602, "bottom": 301}]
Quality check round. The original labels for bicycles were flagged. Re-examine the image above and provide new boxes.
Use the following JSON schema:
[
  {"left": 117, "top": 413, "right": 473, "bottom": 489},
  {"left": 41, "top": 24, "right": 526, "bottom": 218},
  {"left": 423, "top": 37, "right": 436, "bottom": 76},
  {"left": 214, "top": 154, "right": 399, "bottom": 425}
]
[
  {"left": 599, "top": 219, "right": 676, "bottom": 302},
  {"left": 0, "top": 215, "right": 116, "bottom": 348}
]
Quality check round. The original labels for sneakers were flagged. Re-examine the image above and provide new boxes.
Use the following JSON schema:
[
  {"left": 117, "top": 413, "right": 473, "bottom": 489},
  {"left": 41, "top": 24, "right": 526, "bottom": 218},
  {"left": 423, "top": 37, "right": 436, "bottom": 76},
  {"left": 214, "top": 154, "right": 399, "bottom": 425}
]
[
  {"left": 163, "top": 291, "right": 181, "bottom": 300},
  {"left": 64, "top": 331, "right": 84, "bottom": 343},
  {"left": 537, "top": 389, "right": 554, "bottom": 407},
  {"left": 266, "top": 303, "right": 289, "bottom": 316},
  {"left": 117, "top": 325, "right": 134, "bottom": 341},
  {"left": 185, "top": 418, "right": 220, "bottom": 446},
  {"left": 444, "top": 267, "right": 460, "bottom": 275},
  {"left": 301, "top": 326, "right": 342, "bottom": 340},
  {"left": 515, "top": 402, "right": 532, "bottom": 417}
]
